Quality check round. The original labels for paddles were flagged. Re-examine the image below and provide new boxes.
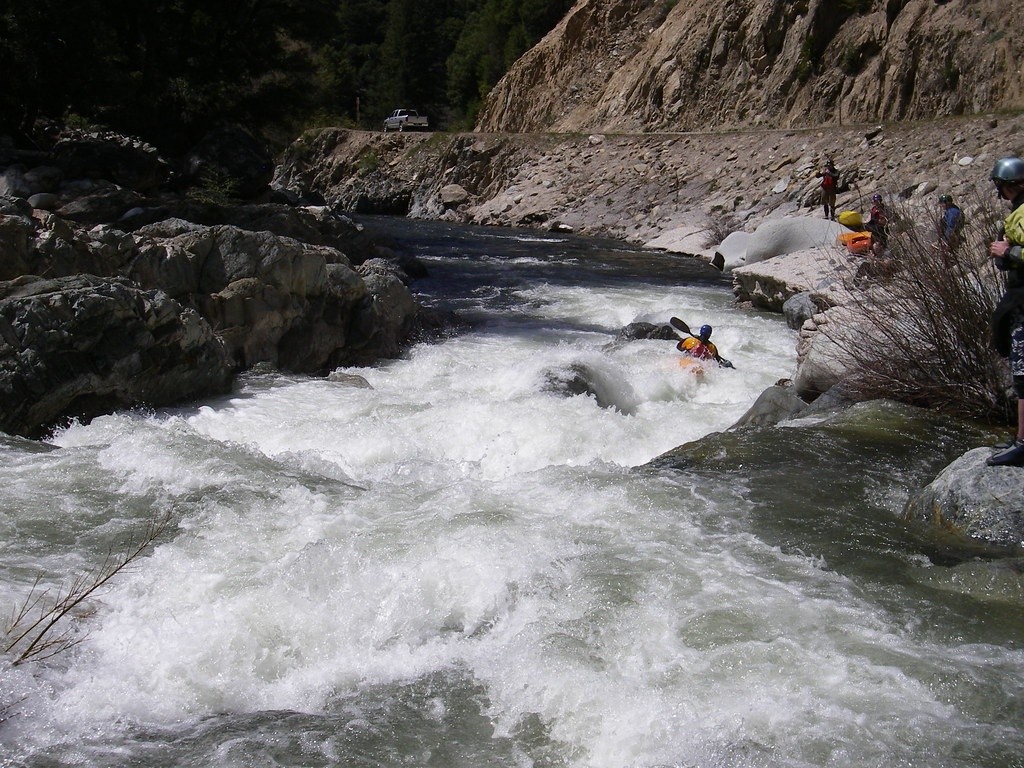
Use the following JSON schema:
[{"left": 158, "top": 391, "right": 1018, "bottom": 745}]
[{"left": 670, "top": 317, "right": 736, "bottom": 370}]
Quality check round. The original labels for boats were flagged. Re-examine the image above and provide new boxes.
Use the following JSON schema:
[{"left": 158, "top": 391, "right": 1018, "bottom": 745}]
[
  {"left": 668, "top": 355, "right": 706, "bottom": 382},
  {"left": 848, "top": 235, "right": 871, "bottom": 253}
]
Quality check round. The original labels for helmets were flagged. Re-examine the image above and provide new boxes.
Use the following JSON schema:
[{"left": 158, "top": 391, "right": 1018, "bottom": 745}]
[
  {"left": 939, "top": 195, "right": 952, "bottom": 203},
  {"left": 700, "top": 325, "right": 712, "bottom": 338},
  {"left": 988, "top": 157, "right": 1024, "bottom": 183},
  {"left": 873, "top": 194, "right": 882, "bottom": 201}
]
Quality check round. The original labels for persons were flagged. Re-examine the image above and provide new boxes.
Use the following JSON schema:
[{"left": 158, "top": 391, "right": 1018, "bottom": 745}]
[
  {"left": 850, "top": 243, "right": 894, "bottom": 291},
  {"left": 865, "top": 194, "right": 887, "bottom": 259},
  {"left": 677, "top": 325, "right": 719, "bottom": 363},
  {"left": 816, "top": 160, "right": 841, "bottom": 221},
  {"left": 986, "top": 156, "right": 1024, "bottom": 465},
  {"left": 935, "top": 195, "right": 965, "bottom": 269}
]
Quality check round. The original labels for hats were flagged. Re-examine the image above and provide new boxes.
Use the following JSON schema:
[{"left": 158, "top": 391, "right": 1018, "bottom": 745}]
[{"left": 824, "top": 160, "right": 835, "bottom": 167}]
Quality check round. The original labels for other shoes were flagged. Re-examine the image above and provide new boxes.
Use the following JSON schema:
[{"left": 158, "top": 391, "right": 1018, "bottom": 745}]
[
  {"left": 831, "top": 217, "right": 834, "bottom": 220},
  {"left": 987, "top": 440, "right": 1024, "bottom": 467},
  {"left": 824, "top": 217, "right": 828, "bottom": 219}
]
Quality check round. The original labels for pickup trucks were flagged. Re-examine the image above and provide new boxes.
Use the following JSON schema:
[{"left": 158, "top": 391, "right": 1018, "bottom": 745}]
[{"left": 383, "top": 108, "right": 428, "bottom": 132}]
